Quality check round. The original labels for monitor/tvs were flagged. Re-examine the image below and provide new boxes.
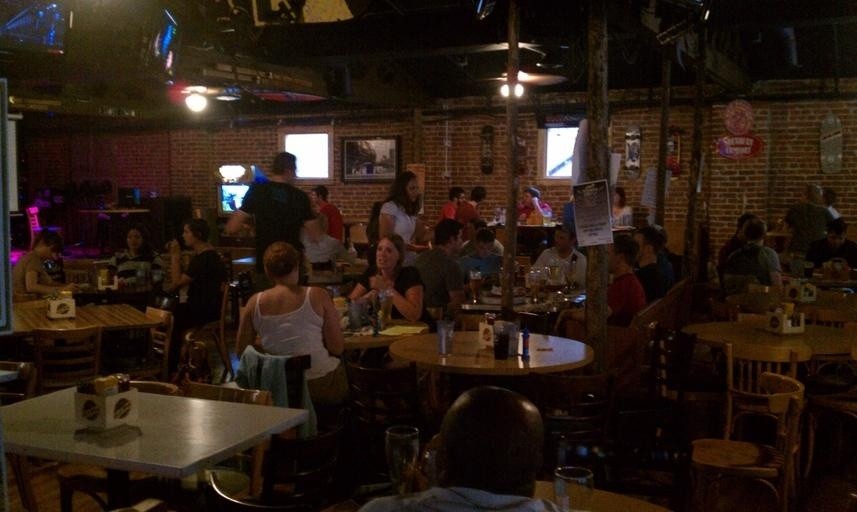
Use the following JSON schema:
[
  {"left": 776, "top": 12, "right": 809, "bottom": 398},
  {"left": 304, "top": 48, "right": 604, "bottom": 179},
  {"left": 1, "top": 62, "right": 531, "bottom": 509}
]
[{"left": 220, "top": 184, "right": 250, "bottom": 213}]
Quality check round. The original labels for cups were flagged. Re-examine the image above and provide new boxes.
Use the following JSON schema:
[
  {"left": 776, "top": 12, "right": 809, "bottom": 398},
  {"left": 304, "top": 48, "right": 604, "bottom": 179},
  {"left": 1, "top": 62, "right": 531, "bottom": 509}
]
[
  {"left": 543, "top": 209, "right": 553, "bottom": 228},
  {"left": 436, "top": 320, "right": 455, "bottom": 355},
  {"left": 344, "top": 299, "right": 368, "bottom": 333},
  {"left": 493, "top": 329, "right": 511, "bottom": 362},
  {"left": 378, "top": 289, "right": 393, "bottom": 328},
  {"left": 553, "top": 467, "right": 594, "bottom": 512}
]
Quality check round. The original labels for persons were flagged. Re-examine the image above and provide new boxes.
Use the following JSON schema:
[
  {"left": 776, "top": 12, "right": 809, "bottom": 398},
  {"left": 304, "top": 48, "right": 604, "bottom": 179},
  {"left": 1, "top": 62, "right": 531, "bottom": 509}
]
[
  {"left": 224, "top": 151, "right": 324, "bottom": 286},
  {"left": 456, "top": 186, "right": 488, "bottom": 225},
  {"left": 349, "top": 231, "right": 438, "bottom": 334},
  {"left": 107, "top": 223, "right": 168, "bottom": 287},
  {"left": 613, "top": 187, "right": 633, "bottom": 227},
  {"left": 729, "top": 219, "right": 783, "bottom": 293},
  {"left": 817, "top": 187, "right": 845, "bottom": 233},
  {"left": 415, "top": 218, "right": 469, "bottom": 324},
  {"left": 236, "top": 240, "right": 347, "bottom": 405},
  {"left": 367, "top": 182, "right": 392, "bottom": 265},
  {"left": 515, "top": 188, "right": 553, "bottom": 225},
  {"left": 359, "top": 385, "right": 565, "bottom": 512},
  {"left": 532, "top": 225, "right": 586, "bottom": 285},
  {"left": 632, "top": 226, "right": 674, "bottom": 302},
  {"left": 778, "top": 184, "right": 835, "bottom": 254},
  {"left": 438, "top": 186, "right": 466, "bottom": 220},
  {"left": 303, "top": 211, "right": 345, "bottom": 271},
  {"left": 380, "top": 170, "right": 428, "bottom": 267},
  {"left": 805, "top": 219, "right": 857, "bottom": 271},
  {"left": 362, "top": 143, "right": 379, "bottom": 174},
  {"left": 717, "top": 215, "right": 757, "bottom": 262},
  {"left": 554, "top": 235, "right": 646, "bottom": 342},
  {"left": 460, "top": 228, "right": 504, "bottom": 278},
  {"left": 11, "top": 227, "right": 84, "bottom": 303},
  {"left": 311, "top": 184, "right": 344, "bottom": 242},
  {"left": 171, "top": 218, "right": 226, "bottom": 351}
]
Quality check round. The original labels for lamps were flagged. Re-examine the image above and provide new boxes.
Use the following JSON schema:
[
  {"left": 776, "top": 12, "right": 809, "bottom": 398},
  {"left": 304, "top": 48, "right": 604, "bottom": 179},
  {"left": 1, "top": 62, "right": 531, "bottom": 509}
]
[{"left": 185, "top": 91, "right": 207, "bottom": 112}]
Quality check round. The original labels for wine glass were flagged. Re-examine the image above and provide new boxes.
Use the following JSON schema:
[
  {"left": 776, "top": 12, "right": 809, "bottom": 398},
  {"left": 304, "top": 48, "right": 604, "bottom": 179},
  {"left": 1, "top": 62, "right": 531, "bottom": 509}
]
[
  {"left": 468, "top": 270, "right": 481, "bottom": 305},
  {"left": 384, "top": 425, "right": 418, "bottom": 511},
  {"left": 528, "top": 270, "right": 546, "bottom": 304},
  {"left": 562, "top": 262, "right": 576, "bottom": 296},
  {"left": 548, "top": 259, "right": 562, "bottom": 292}
]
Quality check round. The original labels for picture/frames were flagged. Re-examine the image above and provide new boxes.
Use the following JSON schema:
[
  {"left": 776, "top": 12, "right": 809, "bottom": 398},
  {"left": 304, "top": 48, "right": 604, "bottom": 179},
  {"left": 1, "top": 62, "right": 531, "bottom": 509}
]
[{"left": 342, "top": 135, "right": 401, "bottom": 182}]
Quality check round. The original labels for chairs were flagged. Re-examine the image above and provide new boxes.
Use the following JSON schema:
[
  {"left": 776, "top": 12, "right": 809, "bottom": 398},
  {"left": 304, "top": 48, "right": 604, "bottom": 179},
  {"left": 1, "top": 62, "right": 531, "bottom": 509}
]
[
  {"left": 33, "top": 325, "right": 100, "bottom": 396},
  {"left": 223, "top": 251, "right": 233, "bottom": 283},
  {"left": 342, "top": 354, "right": 431, "bottom": 445},
  {"left": 237, "top": 345, "right": 351, "bottom": 510},
  {"left": 229, "top": 284, "right": 239, "bottom": 329},
  {"left": 0, "top": 360, "right": 37, "bottom": 511},
  {"left": 676, "top": 322, "right": 857, "bottom": 355},
  {"left": 147, "top": 307, "right": 175, "bottom": 379},
  {"left": 805, "top": 308, "right": 851, "bottom": 328},
  {"left": 239, "top": 271, "right": 253, "bottom": 306},
  {"left": 805, "top": 389, "right": 857, "bottom": 475},
  {"left": 158, "top": 382, "right": 274, "bottom": 511},
  {"left": 58, "top": 381, "right": 183, "bottom": 511},
  {"left": 690, "top": 372, "right": 805, "bottom": 511},
  {"left": 188, "top": 282, "right": 236, "bottom": 378},
  {"left": 726, "top": 340, "right": 799, "bottom": 438},
  {"left": 207, "top": 471, "right": 310, "bottom": 511}
]
